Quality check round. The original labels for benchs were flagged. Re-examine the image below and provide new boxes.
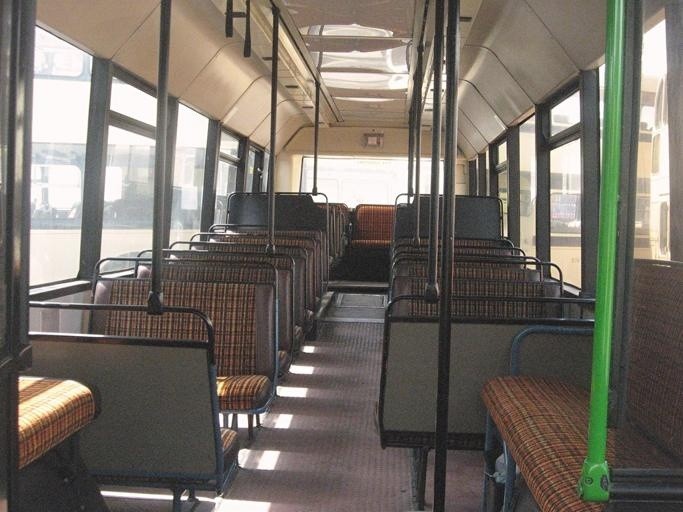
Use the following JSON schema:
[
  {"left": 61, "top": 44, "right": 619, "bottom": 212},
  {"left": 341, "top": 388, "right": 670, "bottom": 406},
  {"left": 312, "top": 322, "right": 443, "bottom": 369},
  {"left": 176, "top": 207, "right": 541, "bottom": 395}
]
[
  {"left": 93, "top": 193, "right": 347, "bottom": 437},
  {"left": 350, "top": 200, "right": 392, "bottom": 271},
  {"left": 2, "top": 369, "right": 107, "bottom": 508},
  {"left": 374, "top": 192, "right": 599, "bottom": 509},
  {"left": 29, "top": 301, "right": 242, "bottom": 510},
  {"left": 485, "top": 258, "right": 683, "bottom": 510}
]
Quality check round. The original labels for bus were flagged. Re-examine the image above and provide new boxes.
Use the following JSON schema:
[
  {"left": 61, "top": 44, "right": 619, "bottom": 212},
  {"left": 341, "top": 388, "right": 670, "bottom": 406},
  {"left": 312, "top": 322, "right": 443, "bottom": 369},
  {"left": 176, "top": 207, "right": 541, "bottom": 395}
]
[{"left": 526, "top": 189, "right": 650, "bottom": 244}]
